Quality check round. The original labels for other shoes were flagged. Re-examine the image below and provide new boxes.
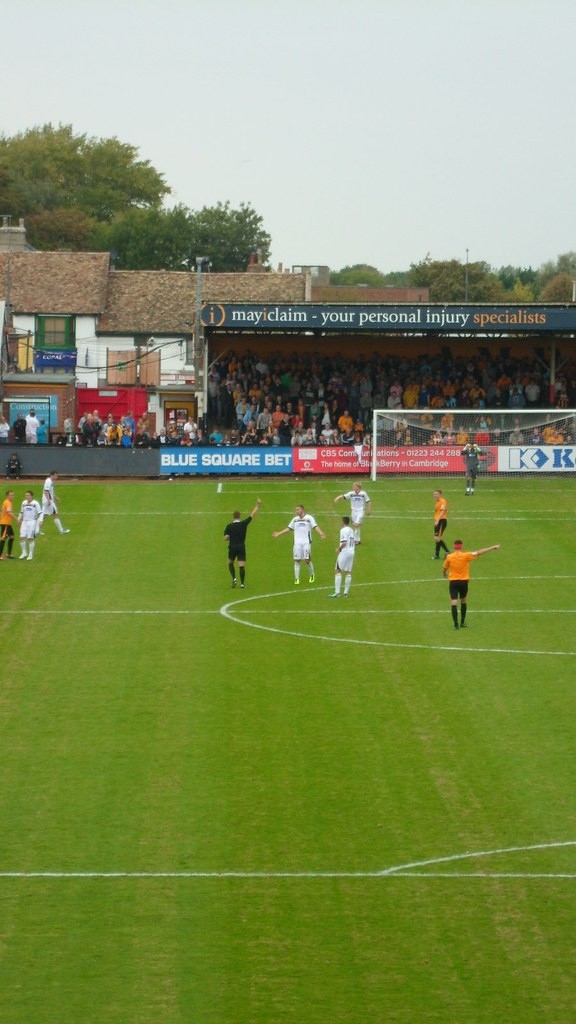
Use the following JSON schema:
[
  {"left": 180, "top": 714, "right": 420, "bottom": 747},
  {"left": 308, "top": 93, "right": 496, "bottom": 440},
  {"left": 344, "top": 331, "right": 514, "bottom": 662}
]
[
  {"left": 453, "top": 626, "right": 459, "bottom": 630},
  {"left": 460, "top": 623, "right": 467, "bottom": 627}
]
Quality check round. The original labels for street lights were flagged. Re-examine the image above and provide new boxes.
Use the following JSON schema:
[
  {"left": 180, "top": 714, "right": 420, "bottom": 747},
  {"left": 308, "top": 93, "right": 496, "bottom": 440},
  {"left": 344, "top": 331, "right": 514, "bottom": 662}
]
[{"left": 193, "top": 256, "right": 207, "bottom": 394}]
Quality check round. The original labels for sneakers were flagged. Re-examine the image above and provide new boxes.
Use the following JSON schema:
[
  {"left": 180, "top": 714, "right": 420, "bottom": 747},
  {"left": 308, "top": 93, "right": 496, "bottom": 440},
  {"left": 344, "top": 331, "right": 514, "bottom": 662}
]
[
  {"left": 327, "top": 593, "right": 341, "bottom": 599},
  {"left": 232, "top": 579, "right": 237, "bottom": 588},
  {"left": 308, "top": 575, "right": 314, "bottom": 583},
  {"left": 355, "top": 541, "right": 361, "bottom": 545},
  {"left": 59, "top": 529, "right": 71, "bottom": 535},
  {"left": 35, "top": 532, "right": 45, "bottom": 536},
  {"left": 430, "top": 555, "right": 440, "bottom": 559},
  {"left": 465, "top": 487, "right": 474, "bottom": 495},
  {"left": 18, "top": 553, "right": 27, "bottom": 560},
  {"left": 0, "top": 557, "right": 4, "bottom": 560},
  {"left": 240, "top": 584, "right": 245, "bottom": 588},
  {"left": 26, "top": 555, "right": 33, "bottom": 561},
  {"left": 343, "top": 593, "right": 348, "bottom": 599},
  {"left": 294, "top": 579, "right": 300, "bottom": 585},
  {"left": 6, "top": 554, "right": 13, "bottom": 558}
]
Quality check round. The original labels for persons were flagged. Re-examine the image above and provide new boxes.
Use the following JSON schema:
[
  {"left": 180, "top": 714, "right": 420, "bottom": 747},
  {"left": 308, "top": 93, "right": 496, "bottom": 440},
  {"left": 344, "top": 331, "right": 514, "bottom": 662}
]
[
  {"left": 0, "top": 416, "right": 10, "bottom": 444},
  {"left": 0, "top": 490, "right": 21, "bottom": 561},
  {"left": 17, "top": 491, "right": 43, "bottom": 560},
  {"left": 206, "top": 349, "right": 576, "bottom": 445},
  {"left": 25, "top": 409, "right": 40, "bottom": 443},
  {"left": 104, "top": 413, "right": 122, "bottom": 446},
  {"left": 64, "top": 415, "right": 75, "bottom": 444},
  {"left": 334, "top": 481, "right": 371, "bottom": 545},
  {"left": 443, "top": 539, "right": 501, "bottom": 630},
  {"left": 328, "top": 517, "right": 355, "bottom": 597},
  {"left": 430, "top": 490, "right": 451, "bottom": 559},
  {"left": 460, "top": 443, "right": 483, "bottom": 496},
  {"left": 123, "top": 410, "right": 134, "bottom": 434},
  {"left": 121, "top": 428, "right": 132, "bottom": 447},
  {"left": 5, "top": 451, "right": 23, "bottom": 480},
  {"left": 36, "top": 420, "right": 47, "bottom": 443},
  {"left": 38, "top": 470, "right": 70, "bottom": 535},
  {"left": 157, "top": 416, "right": 224, "bottom": 446},
  {"left": 78, "top": 410, "right": 104, "bottom": 447},
  {"left": 271, "top": 504, "right": 326, "bottom": 584},
  {"left": 133, "top": 412, "right": 150, "bottom": 448},
  {"left": 150, "top": 432, "right": 161, "bottom": 448},
  {"left": 13, "top": 412, "right": 27, "bottom": 443},
  {"left": 223, "top": 498, "right": 262, "bottom": 589}
]
